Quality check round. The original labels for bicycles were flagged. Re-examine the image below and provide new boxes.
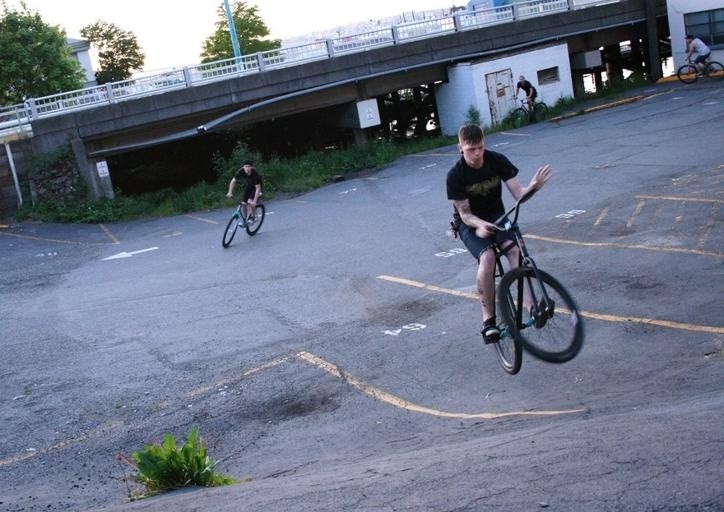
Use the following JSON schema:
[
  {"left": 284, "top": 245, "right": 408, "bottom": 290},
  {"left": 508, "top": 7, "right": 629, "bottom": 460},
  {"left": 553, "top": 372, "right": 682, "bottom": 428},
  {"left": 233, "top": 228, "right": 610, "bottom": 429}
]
[
  {"left": 222, "top": 193, "right": 265, "bottom": 248},
  {"left": 483, "top": 188, "right": 585, "bottom": 375},
  {"left": 677, "top": 54, "right": 724, "bottom": 84},
  {"left": 512, "top": 97, "right": 548, "bottom": 128}
]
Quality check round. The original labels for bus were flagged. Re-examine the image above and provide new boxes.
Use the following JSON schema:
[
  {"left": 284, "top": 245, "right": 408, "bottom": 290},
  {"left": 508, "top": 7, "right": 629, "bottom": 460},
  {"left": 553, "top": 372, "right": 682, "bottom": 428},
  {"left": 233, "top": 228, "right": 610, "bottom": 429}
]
[{"left": 467, "top": 0, "right": 510, "bottom": 19}]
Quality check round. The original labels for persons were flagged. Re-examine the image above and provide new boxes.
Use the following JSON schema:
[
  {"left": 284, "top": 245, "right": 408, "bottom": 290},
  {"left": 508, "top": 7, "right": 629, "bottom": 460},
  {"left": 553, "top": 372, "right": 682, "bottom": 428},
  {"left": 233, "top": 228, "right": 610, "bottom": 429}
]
[
  {"left": 685, "top": 34, "right": 711, "bottom": 79},
  {"left": 226, "top": 160, "right": 262, "bottom": 228},
  {"left": 444, "top": 123, "right": 551, "bottom": 346},
  {"left": 513, "top": 76, "right": 537, "bottom": 115}
]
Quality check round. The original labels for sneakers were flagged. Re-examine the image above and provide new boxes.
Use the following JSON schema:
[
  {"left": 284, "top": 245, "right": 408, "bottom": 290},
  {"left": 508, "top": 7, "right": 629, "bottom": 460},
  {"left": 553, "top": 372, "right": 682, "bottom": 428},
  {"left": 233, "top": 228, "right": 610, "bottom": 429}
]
[
  {"left": 481, "top": 324, "right": 500, "bottom": 338},
  {"left": 530, "top": 297, "right": 554, "bottom": 328},
  {"left": 238, "top": 216, "right": 255, "bottom": 226}
]
